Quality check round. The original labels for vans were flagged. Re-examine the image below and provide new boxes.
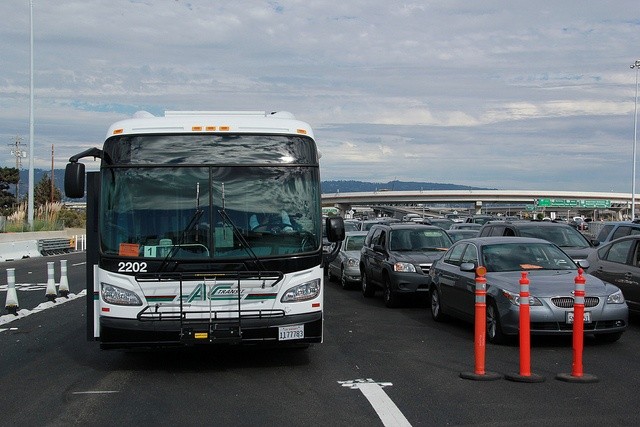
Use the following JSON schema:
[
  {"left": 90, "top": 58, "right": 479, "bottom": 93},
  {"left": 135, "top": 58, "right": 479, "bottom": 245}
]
[{"left": 474, "top": 221, "right": 600, "bottom": 267}]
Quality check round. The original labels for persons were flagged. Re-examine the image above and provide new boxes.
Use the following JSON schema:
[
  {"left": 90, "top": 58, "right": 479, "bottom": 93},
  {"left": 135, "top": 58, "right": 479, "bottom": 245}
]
[{"left": 249, "top": 192, "right": 293, "bottom": 237}]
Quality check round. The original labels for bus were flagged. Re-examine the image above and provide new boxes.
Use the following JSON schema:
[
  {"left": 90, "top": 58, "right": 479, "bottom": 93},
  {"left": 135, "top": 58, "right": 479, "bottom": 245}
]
[{"left": 63, "top": 106, "right": 346, "bottom": 351}]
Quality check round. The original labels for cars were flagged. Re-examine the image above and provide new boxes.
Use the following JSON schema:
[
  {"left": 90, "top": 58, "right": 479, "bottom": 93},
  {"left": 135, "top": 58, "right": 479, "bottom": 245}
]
[
  {"left": 328, "top": 230, "right": 379, "bottom": 289},
  {"left": 428, "top": 236, "right": 630, "bottom": 348},
  {"left": 580, "top": 233, "right": 640, "bottom": 309},
  {"left": 322, "top": 211, "right": 530, "bottom": 241}
]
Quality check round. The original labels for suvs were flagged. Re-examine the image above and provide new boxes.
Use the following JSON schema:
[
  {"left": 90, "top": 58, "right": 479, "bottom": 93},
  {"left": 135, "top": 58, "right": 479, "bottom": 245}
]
[
  {"left": 595, "top": 219, "right": 640, "bottom": 244},
  {"left": 359, "top": 221, "right": 456, "bottom": 307}
]
